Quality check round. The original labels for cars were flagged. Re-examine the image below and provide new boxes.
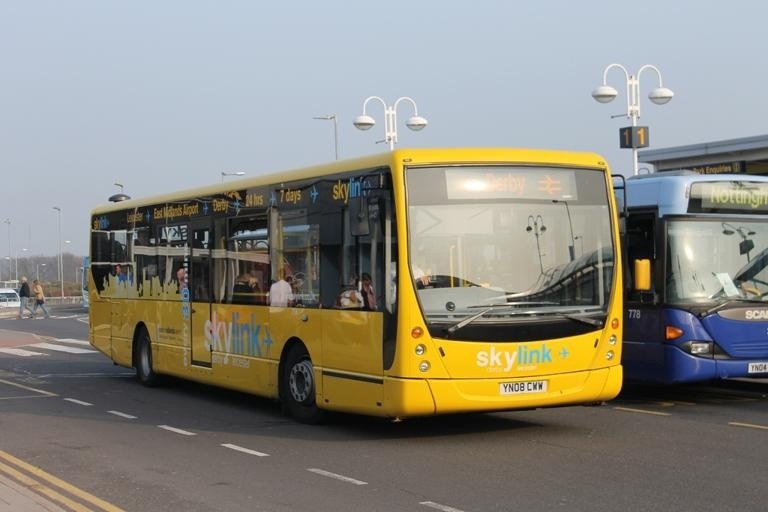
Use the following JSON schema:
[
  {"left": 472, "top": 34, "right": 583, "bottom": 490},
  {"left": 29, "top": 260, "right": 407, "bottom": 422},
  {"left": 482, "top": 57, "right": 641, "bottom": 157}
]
[{"left": 0, "top": 287, "right": 21, "bottom": 308}]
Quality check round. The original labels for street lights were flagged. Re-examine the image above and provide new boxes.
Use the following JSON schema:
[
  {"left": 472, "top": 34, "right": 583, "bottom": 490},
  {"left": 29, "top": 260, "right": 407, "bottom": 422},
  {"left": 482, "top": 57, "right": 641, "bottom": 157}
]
[
  {"left": 312, "top": 113, "right": 338, "bottom": 160},
  {"left": 221, "top": 172, "right": 245, "bottom": 184},
  {"left": 592, "top": 62, "right": 674, "bottom": 175},
  {"left": 353, "top": 95, "right": 428, "bottom": 150},
  {"left": 2, "top": 206, "right": 72, "bottom": 304},
  {"left": 114, "top": 183, "right": 138, "bottom": 197}
]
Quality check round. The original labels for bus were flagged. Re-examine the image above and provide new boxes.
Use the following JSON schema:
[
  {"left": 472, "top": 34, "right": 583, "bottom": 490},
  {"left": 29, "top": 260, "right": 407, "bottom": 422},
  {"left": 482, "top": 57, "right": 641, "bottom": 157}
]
[
  {"left": 87, "top": 147, "right": 625, "bottom": 425},
  {"left": 613, "top": 168, "right": 766, "bottom": 386},
  {"left": 82, "top": 257, "right": 91, "bottom": 309}
]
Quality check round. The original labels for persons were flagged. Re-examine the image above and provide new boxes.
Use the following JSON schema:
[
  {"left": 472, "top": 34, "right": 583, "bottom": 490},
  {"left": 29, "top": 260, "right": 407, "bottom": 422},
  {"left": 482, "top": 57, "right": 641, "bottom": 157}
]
[
  {"left": 31, "top": 280, "right": 50, "bottom": 319},
  {"left": 390, "top": 260, "right": 431, "bottom": 313},
  {"left": 135, "top": 231, "right": 152, "bottom": 246},
  {"left": 361, "top": 272, "right": 375, "bottom": 311},
  {"left": 265, "top": 267, "right": 294, "bottom": 335},
  {"left": 19, "top": 276, "right": 38, "bottom": 319},
  {"left": 115, "top": 264, "right": 125, "bottom": 281}
]
